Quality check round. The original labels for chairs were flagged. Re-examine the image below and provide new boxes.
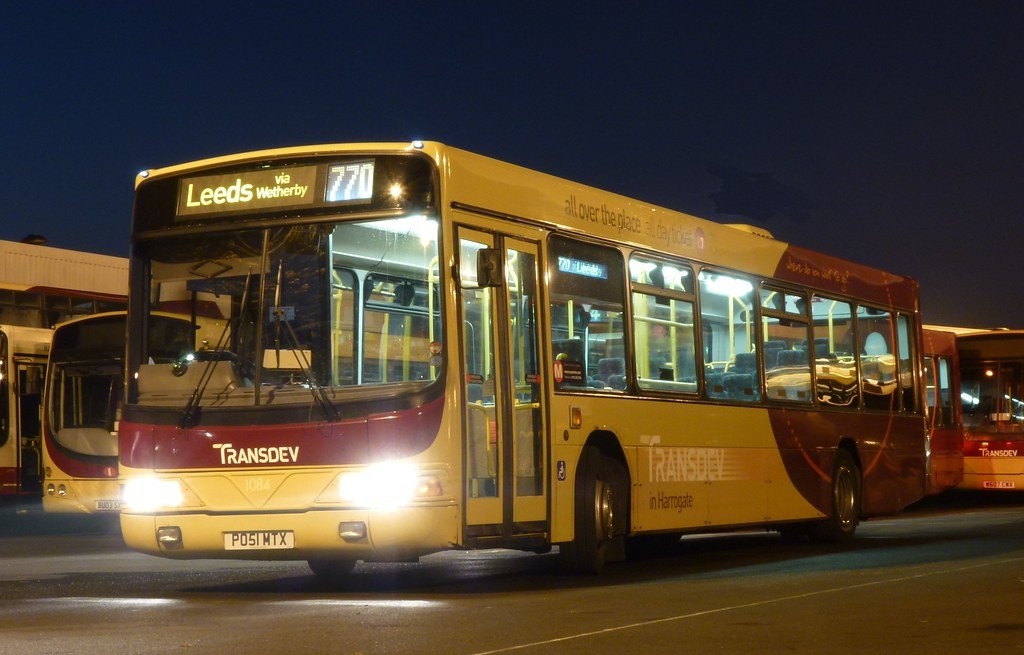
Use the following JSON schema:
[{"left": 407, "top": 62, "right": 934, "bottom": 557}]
[{"left": 564, "top": 339, "right": 912, "bottom": 408}]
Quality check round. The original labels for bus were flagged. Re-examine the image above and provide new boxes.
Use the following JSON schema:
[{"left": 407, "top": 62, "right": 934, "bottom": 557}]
[
  {"left": 923, "top": 323, "right": 1024, "bottom": 513},
  {"left": 0, "top": 322, "right": 79, "bottom": 504},
  {"left": 33, "top": 307, "right": 444, "bottom": 533},
  {"left": 110, "top": 140, "right": 935, "bottom": 587}
]
